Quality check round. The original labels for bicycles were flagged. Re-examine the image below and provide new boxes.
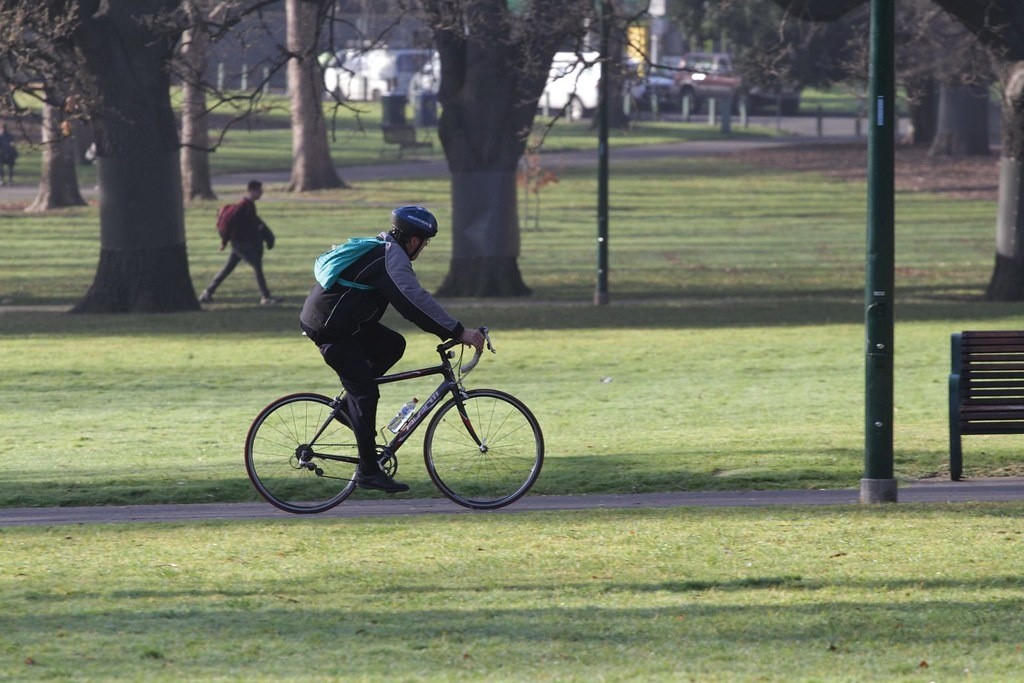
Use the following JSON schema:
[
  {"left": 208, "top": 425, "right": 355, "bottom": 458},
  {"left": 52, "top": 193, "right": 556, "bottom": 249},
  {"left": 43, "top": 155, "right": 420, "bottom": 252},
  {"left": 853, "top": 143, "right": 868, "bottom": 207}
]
[{"left": 244, "top": 327, "right": 545, "bottom": 514}]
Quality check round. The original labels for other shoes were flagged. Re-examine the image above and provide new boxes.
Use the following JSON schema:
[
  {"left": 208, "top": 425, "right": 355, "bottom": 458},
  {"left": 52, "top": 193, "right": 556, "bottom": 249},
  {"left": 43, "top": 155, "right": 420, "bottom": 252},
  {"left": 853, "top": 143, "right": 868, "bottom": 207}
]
[
  {"left": 357, "top": 470, "right": 410, "bottom": 492},
  {"left": 330, "top": 401, "right": 377, "bottom": 437},
  {"left": 261, "top": 297, "right": 283, "bottom": 304},
  {"left": 199, "top": 290, "right": 213, "bottom": 303}
]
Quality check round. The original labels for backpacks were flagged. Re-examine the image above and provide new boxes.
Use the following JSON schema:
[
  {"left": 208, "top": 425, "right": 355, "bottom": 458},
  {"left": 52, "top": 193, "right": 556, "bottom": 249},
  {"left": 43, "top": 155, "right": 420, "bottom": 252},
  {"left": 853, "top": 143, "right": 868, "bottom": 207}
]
[
  {"left": 314, "top": 237, "right": 387, "bottom": 290},
  {"left": 216, "top": 204, "right": 239, "bottom": 251}
]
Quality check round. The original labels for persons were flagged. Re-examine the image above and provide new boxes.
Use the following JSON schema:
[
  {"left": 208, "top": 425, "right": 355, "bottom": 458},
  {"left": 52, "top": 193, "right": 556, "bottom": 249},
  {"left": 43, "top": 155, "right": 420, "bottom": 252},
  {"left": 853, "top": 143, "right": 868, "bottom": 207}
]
[
  {"left": 0, "top": 124, "right": 18, "bottom": 185},
  {"left": 300, "top": 205, "right": 485, "bottom": 494},
  {"left": 198, "top": 180, "right": 284, "bottom": 304}
]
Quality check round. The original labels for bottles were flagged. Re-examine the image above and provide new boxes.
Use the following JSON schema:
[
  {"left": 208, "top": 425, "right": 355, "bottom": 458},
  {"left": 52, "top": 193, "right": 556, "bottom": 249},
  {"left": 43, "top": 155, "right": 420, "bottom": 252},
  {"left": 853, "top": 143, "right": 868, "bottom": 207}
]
[{"left": 387, "top": 398, "right": 418, "bottom": 434}]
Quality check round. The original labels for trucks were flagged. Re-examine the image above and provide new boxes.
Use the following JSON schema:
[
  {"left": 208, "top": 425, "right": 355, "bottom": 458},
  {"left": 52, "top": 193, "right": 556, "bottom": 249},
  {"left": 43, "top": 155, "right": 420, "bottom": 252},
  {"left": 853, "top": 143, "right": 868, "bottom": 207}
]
[
  {"left": 323, "top": 48, "right": 435, "bottom": 102},
  {"left": 537, "top": 51, "right": 601, "bottom": 120}
]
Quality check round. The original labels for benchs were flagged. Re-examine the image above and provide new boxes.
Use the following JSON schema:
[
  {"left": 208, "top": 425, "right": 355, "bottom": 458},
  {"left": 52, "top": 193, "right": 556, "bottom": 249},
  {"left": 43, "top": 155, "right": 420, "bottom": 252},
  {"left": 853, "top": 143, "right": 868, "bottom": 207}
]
[
  {"left": 949, "top": 330, "right": 1024, "bottom": 481},
  {"left": 378, "top": 124, "right": 435, "bottom": 158}
]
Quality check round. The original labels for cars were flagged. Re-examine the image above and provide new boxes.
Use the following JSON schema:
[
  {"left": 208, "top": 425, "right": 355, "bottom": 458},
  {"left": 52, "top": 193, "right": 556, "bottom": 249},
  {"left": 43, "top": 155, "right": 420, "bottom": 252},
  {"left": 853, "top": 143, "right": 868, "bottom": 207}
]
[
  {"left": 628, "top": 55, "right": 680, "bottom": 109},
  {"left": 673, "top": 52, "right": 798, "bottom": 116}
]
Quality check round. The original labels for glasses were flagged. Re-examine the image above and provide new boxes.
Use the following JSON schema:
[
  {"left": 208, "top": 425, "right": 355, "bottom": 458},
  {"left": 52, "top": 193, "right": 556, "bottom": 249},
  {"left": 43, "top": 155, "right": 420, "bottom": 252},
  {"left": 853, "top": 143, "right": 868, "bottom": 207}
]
[{"left": 425, "top": 238, "right": 432, "bottom": 245}]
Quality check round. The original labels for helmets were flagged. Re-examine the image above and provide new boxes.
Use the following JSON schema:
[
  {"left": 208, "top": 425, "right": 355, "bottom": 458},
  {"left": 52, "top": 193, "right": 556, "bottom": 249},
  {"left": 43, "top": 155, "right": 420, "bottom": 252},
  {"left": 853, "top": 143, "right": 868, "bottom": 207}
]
[{"left": 392, "top": 206, "right": 438, "bottom": 237}]
